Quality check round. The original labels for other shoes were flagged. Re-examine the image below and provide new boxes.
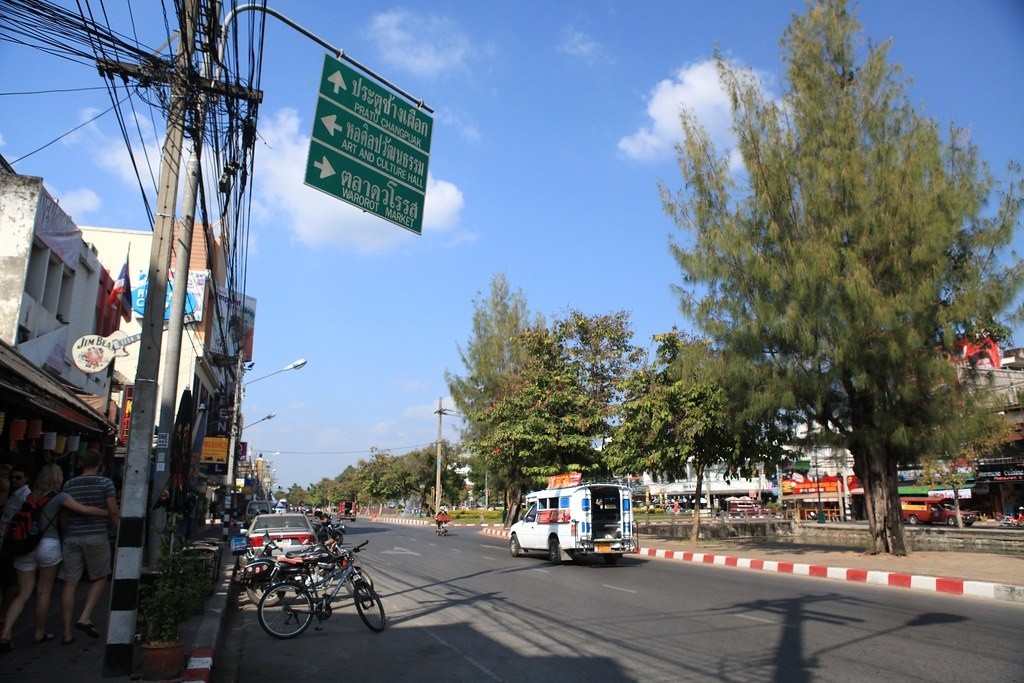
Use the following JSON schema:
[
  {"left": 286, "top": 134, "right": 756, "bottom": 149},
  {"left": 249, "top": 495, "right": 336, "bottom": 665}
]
[{"left": 435, "top": 529, "right": 438, "bottom": 533}]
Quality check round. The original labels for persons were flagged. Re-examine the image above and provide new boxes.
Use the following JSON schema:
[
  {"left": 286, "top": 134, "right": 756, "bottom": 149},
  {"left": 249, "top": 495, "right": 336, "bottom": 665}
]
[
  {"left": 435, "top": 506, "right": 449, "bottom": 532},
  {"left": 0, "top": 449, "right": 121, "bottom": 650}
]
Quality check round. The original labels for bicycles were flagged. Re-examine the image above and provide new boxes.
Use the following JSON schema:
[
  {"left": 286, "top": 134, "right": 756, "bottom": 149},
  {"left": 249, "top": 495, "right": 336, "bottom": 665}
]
[{"left": 256, "top": 537, "right": 385, "bottom": 639}]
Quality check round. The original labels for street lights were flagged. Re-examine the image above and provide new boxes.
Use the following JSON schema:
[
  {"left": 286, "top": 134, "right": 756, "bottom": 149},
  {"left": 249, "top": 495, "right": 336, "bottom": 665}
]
[{"left": 221, "top": 357, "right": 308, "bottom": 547}]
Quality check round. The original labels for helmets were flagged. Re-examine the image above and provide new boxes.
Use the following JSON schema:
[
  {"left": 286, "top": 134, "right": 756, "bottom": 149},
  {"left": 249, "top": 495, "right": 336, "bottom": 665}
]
[{"left": 439, "top": 506, "right": 445, "bottom": 510}]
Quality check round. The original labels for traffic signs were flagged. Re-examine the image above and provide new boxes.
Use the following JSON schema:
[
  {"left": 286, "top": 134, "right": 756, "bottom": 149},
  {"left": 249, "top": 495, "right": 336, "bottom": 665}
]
[{"left": 300, "top": 52, "right": 435, "bottom": 236}]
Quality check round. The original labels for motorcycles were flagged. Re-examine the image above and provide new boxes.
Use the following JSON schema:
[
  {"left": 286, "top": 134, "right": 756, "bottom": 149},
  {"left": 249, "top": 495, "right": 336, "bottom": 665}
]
[{"left": 230, "top": 529, "right": 372, "bottom": 606}]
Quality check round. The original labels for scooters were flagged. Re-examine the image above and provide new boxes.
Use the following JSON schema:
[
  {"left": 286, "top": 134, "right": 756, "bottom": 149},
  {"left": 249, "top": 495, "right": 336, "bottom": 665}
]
[
  {"left": 433, "top": 515, "right": 451, "bottom": 536},
  {"left": 999, "top": 506, "right": 1024, "bottom": 528}
]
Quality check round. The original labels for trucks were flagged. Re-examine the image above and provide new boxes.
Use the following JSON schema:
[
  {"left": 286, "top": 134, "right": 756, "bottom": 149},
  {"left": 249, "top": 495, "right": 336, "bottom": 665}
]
[
  {"left": 508, "top": 480, "right": 635, "bottom": 565},
  {"left": 339, "top": 501, "right": 357, "bottom": 523}
]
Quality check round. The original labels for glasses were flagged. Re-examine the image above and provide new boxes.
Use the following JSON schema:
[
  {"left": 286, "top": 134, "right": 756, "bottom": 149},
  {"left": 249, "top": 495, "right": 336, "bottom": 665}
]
[{"left": 9, "top": 474, "right": 23, "bottom": 480}]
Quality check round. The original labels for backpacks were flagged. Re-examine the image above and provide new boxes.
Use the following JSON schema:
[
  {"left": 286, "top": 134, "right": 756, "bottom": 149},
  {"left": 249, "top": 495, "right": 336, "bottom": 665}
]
[{"left": 2, "top": 489, "right": 68, "bottom": 556}]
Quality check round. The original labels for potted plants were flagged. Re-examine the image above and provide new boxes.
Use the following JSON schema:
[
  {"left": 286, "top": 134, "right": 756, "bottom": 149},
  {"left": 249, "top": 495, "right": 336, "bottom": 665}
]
[{"left": 127, "top": 513, "right": 216, "bottom": 680}]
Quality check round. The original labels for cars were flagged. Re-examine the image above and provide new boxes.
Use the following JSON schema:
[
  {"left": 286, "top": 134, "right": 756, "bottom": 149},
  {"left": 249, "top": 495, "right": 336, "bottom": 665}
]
[
  {"left": 238, "top": 512, "right": 320, "bottom": 565},
  {"left": 272, "top": 498, "right": 313, "bottom": 514}
]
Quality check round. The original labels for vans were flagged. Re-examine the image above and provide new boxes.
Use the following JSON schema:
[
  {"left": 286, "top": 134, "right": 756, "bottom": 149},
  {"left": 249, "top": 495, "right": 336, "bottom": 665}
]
[
  {"left": 899, "top": 496, "right": 978, "bottom": 526},
  {"left": 244, "top": 500, "right": 271, "bottom": 526}
]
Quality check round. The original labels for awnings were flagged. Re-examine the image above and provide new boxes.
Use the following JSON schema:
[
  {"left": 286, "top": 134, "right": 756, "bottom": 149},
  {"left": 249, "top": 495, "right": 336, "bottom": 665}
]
[{"left": 0, "top": 339, "right": 116, "bottom": 431}]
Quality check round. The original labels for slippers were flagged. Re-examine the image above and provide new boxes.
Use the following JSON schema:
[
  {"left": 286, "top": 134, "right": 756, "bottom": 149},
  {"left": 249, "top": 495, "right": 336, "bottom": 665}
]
[
  {"left": 62, "top": 633, "right": 77, "bottom": 645},
  {"left": 75, "top": 624, "right": 100, "bottom": 639},
  {"left": 33, "top": 632, "right": 57, "bottom": 644},
  {"left": 0, "top": 638, "right": 16, "bottom": 653}
]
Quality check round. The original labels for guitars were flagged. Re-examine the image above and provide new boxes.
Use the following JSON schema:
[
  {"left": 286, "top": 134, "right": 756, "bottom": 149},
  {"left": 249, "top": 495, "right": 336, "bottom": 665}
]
[{"left": 72, "top": 313, "right": 196, "bottom": 374}]
